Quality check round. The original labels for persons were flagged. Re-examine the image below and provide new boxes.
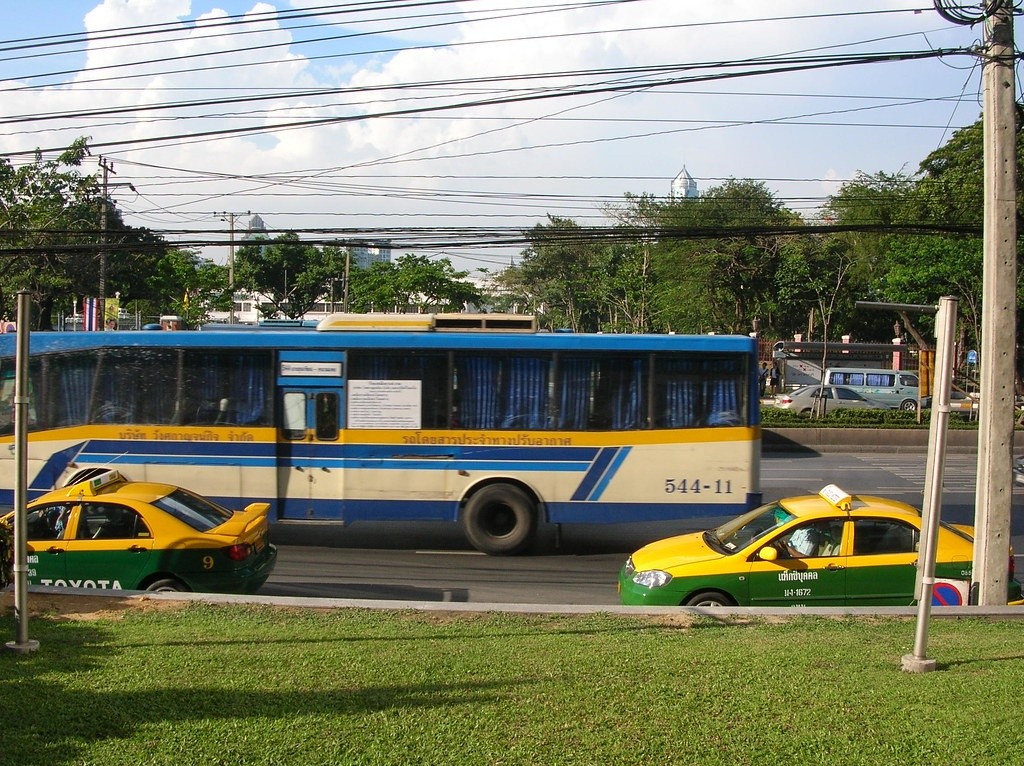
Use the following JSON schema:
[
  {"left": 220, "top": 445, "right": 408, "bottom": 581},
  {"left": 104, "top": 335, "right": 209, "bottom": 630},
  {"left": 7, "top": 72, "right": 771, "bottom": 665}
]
[
  {"left": 41, "top": 509, "right": 60, "bottom": 538},
  {"left": 104, "top": 318, "right": 115, "bottom": 331},
  {"left": 778, "top": 527, "right": 820, "bottom": 557},
  {"left": 759, "top": 362, "right": 768, "bottom": 397},
  {"left": 770, "top": 362, "right": 783, "bottom": 395}
]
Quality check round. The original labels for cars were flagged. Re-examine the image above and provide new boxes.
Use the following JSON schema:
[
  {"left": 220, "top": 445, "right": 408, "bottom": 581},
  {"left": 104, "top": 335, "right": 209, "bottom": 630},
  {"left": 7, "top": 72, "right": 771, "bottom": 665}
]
[
  {"left": 615, "top": 484, "right": 1024, "bottom": 607},
  {"left": 771, "top": 385, "right": 892, "bottom": 420},
  {"left": 0, "top": 469, "right": 277, "bottom": 595}
]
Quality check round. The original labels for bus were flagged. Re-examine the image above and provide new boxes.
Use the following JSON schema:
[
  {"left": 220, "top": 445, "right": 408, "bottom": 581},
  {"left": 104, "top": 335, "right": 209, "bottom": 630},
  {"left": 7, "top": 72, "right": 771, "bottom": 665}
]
[{"left": 0, "top": 309, "right": 763, "bottom": 561}]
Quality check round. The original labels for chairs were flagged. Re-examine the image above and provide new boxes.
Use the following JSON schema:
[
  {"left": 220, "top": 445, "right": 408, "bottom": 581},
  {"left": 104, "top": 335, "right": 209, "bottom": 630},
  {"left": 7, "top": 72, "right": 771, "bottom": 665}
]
[
  {"left": 818, "top": 525, "right": 842, "bottom": 557},
  {"left": 212, "top": 396, "right": 235, "bottom": 425},
  {"left": 92, "top": 509, "right": 125, "bottom": 539}
]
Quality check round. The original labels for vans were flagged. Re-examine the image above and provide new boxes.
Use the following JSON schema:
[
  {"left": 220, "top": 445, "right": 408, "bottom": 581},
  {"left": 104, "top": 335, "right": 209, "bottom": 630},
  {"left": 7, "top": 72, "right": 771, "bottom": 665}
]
[{"left": 822, "top": 366, "right": 927, "bottom": 411}]
